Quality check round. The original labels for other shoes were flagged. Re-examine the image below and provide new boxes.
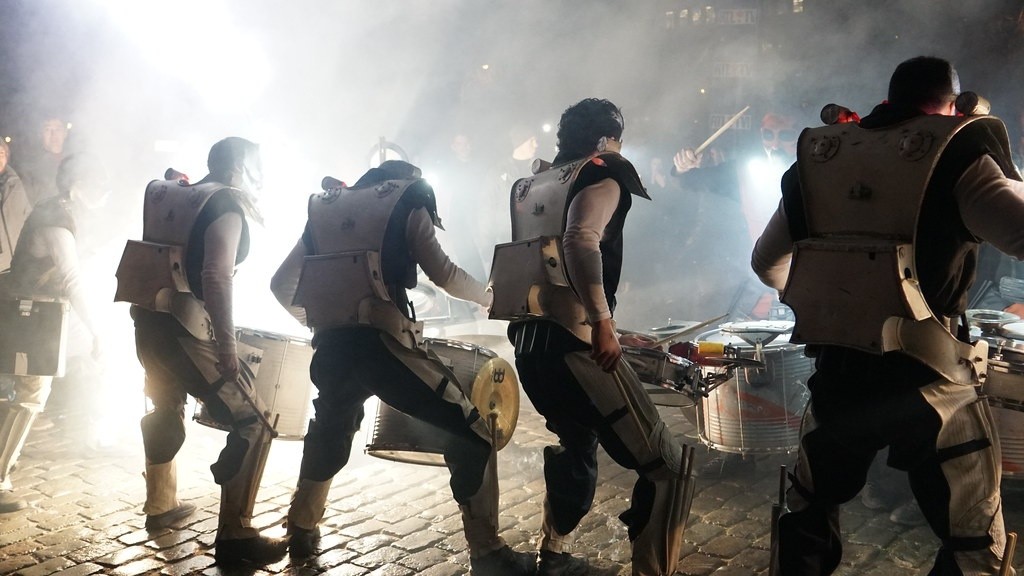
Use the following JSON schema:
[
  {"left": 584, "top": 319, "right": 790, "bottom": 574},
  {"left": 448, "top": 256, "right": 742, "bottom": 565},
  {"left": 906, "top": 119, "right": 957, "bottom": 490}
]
[
  {"left": 215, "top": 538, "right": 289, "bottom": 569},
  {"left": 287, "top": 521, "right": 320, "bottom": 556},
  {"left": 0, "top": 489, "right": 27, "bottom": 512},
  {"left": 146, "top": 503, "right": 197, "bottom": 530},
  {"left": 536, "top": 548, "right": 588, "bottom": 576},
  {"left": 469, "top": 544, "right": 536, "bottom": 576}
]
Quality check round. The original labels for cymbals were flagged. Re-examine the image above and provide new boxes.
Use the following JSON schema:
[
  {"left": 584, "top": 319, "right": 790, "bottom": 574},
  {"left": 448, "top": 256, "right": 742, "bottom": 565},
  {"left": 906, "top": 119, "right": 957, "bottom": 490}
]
[
  {"left": 719, "top": 320, "right": 795, "bottom": 348},
  {"left": 638, "top": 320, "right": 709, "bottom": 338},
  {"left": 469, "top": 358, "right": 520, "bottom": 450},
  {"left": 705, "top": 357, "right": 764, "bottom": 368},
  {"left": 965, "top": 308, "right": 1024, "bottom": 340}
]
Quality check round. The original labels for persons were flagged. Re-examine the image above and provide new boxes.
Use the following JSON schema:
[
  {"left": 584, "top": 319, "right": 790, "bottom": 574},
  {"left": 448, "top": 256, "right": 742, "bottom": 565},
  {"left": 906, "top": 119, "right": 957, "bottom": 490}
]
[
  {"left": 270, "top": 160, "right": 537, "bottom": 576},
  {"left": 129, "top": 136, "right": 289, "bottom": 569},
  {"left": 509, "top": 97, "right": 698, "bottom": 576},
  {"left": 0, "top": 107, "right": 107, "bottom": 513},
  {"left": 675, "top": 93, "right": 810, "bottom": 321},
  {"left": 750, "top": 56, "right": 1024, "bottom": 576}
]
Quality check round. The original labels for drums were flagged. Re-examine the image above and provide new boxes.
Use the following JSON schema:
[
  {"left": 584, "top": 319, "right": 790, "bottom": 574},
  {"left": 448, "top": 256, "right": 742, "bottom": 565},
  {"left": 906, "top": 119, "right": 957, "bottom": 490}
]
[
  {"left": 620, "top": 345, "right": 702, "bottom": 408},
  {"left": 697, "top": 327, "right": 819, "bottom": 456},
  {"left": 364, "top": 336, "right": 499, "bottom": 467},
  {"left": 192, "top": 325, "right": 316, "bottom": 442},
  {"left": 970, "top": 327, "right": 1024, "bottom": 493}
]
